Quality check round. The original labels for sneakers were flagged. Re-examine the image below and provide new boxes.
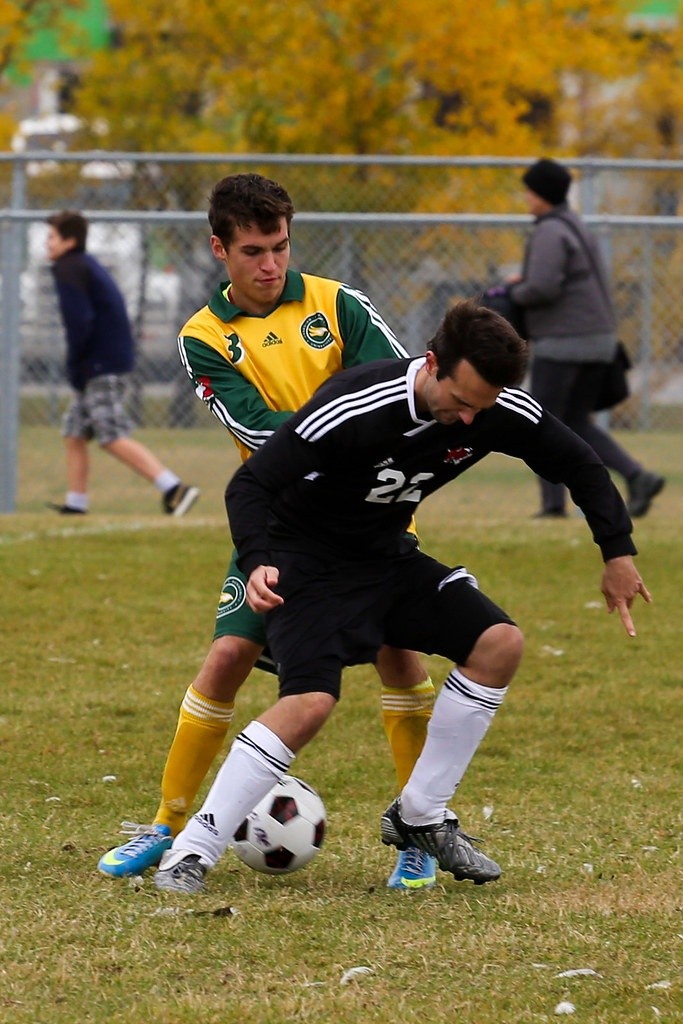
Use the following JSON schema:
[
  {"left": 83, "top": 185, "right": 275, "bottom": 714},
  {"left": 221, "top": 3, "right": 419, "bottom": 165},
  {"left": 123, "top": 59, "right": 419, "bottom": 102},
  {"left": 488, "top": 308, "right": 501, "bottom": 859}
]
[
  {"left": 98, "top": 820, "right": 172, "bottom": 881},
  {"left": 379, "top": 800, "right": 504, "bottom": 885},
  {"left": 153, "top": 844, "right": 209, "bottom": 896},
  {"left": 45, "top": 500, "right": 88, "bottom": 514},
  {"left": 160, "top": 480, "right": 198, "bottom": 515},
  {"left": 387, "top": 843, "right": 437, "bottom": 892}
]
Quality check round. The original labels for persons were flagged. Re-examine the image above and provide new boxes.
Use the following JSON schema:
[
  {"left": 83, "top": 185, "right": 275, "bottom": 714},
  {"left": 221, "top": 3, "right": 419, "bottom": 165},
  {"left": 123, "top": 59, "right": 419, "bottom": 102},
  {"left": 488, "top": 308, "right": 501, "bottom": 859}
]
[
  {"left": 478, "top": 156, "right": 666, "bottom": 520},
  {"left": 155, "top": 297, "right": 653, "bottom": 898},
  {"left": 96, "top": 173, "right": 443, "bottom": 890},
  {"left": 43, "top": 211, "right": 200, "bottom": 518}
]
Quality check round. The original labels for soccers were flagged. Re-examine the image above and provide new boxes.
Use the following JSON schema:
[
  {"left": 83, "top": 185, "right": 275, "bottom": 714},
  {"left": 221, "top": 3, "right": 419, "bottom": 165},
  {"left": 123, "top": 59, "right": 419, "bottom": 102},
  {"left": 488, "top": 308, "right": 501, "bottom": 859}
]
[{"left": 232, "top": 775, "right": 327, "bottom": 876}]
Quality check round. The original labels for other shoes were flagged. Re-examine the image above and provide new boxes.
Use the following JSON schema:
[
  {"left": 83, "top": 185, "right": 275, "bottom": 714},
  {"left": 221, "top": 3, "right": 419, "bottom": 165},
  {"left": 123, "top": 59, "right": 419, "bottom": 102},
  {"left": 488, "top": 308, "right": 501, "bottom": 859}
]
[{"left": 626, "top": 469, "right": 666, "bottom": 518}]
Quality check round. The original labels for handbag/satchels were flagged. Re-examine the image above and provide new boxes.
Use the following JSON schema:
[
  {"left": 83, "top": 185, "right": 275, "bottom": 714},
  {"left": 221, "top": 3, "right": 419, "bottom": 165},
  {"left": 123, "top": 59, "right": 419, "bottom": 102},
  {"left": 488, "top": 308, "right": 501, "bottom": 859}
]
[{"left": 526, "top": 339, "right": 641, "bottom": 420}]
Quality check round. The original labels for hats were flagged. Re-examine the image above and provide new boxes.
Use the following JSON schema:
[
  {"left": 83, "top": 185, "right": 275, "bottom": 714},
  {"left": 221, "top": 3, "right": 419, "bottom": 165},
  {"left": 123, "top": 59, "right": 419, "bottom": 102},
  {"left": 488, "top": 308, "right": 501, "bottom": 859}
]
[{"left": 517, "top": 158, "right": 576, "bottom": 209}]
